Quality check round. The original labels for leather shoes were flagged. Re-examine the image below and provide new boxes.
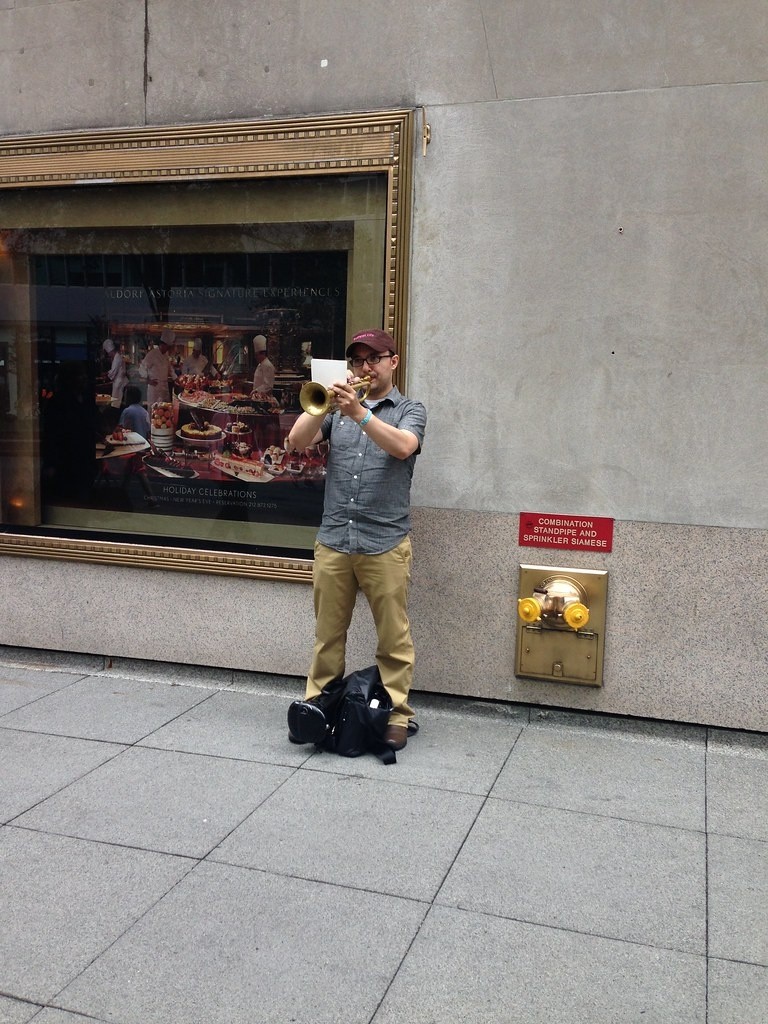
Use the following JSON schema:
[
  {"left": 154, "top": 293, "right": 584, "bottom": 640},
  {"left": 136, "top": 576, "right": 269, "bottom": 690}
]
[
  {"left": 380, "top": 723, "right": 409, "bottom": 750},
  {"left": 288, "top": 728, "right": 308, "bottom": 744}
]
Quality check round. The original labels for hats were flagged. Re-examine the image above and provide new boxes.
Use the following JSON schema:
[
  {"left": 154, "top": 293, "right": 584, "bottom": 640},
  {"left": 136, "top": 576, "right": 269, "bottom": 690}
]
[
  {"left": 253, "top": 335, "right": 266, "bottom": 353},
  {"left": 103, "top": 339, "right": 115, "bottom": 353},
  {"left": 345, "top": 328, "right": 397, "bottom": 359},
  {"left": 193, "top": 338, "right": 202, "bottom": 350},
  {"left": 160, "top": 328, "right": 176, "bottom": 346}
]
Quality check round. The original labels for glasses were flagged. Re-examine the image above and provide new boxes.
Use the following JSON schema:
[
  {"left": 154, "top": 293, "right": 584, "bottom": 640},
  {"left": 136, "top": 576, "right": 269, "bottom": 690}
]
[{"left": 349, "top": 354, "right": 391, "bottom": 367}]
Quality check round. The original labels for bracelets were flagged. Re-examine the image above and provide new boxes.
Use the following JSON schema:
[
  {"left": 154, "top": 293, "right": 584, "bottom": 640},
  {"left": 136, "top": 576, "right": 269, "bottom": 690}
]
[{"left": 357, "top": 409, "right": 372, "bottom": 427}]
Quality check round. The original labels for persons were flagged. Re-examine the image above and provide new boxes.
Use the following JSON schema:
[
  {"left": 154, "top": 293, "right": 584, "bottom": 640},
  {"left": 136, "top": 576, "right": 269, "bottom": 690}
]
[
  {"left": 250, "top": 335, "right": 275, "bottom": 399},
  {"left": 288, "top": 330, "right": 427, "bottom": 751},
  {"left": 101, "top": 385, "right": 160, "bottom": 509},
  {"left": 182, "top": 337, "right": 221, "bottom": 380},
  {"left": 139, "top": 329, "right": 179, "bottom": 413},
  {"left": 103, "top": 339, "right": 129, "bottom": 408}
]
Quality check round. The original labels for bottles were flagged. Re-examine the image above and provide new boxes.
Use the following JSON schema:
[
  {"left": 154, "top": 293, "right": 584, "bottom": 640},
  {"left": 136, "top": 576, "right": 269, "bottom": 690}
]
[{"left": 291, "top": 449, "right": 301, "bottom": 470}]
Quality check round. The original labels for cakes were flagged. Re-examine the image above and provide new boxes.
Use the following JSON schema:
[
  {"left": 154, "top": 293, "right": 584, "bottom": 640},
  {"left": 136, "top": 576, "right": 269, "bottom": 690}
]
[{"left": 181, "top": 411, "right": 223, "bottom": 440}]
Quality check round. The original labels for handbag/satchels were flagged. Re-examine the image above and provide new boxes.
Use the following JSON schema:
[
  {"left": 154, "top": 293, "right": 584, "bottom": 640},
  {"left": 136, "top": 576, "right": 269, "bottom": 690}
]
[{"left": 314, "top": 665, "right": 420, "bottom": 765}]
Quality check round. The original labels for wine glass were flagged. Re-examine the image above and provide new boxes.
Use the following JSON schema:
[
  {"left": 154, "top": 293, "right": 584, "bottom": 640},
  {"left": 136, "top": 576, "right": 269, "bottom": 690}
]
[
  {"left": 306, "top": 445, "right": 316, "bottom": 475},
  {"left": 317, "top": 443, "right": 329, "bottom": 475}
]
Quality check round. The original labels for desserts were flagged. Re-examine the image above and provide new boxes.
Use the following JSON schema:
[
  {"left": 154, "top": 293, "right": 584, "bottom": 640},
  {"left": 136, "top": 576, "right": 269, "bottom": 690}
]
[
  {"left": 113, "top": 424, "right": 124, "bottom": 441},
  {"left": 143, "top": 438, "right": 195, "bottom": 478},
  {"left": 212, "top": 421, "right": 300, "bottom": 478}
]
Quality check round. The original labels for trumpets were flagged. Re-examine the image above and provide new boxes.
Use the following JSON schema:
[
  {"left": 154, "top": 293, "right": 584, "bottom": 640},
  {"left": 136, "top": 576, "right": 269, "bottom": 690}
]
[{"left": 300, "top": 374, "right": 372, "bottom": 416}]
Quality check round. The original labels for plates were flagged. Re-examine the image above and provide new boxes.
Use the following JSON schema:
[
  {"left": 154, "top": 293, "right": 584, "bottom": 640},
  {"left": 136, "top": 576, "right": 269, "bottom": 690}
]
[
  {"left": 268, "top": 466, "right": 286, "bottom": 475},
  {"left": 287, "top": 464, "right": 303, "bottom": 474},
  {"left": 211, "top": 459, "right": 274, "bottom": 482}
]
